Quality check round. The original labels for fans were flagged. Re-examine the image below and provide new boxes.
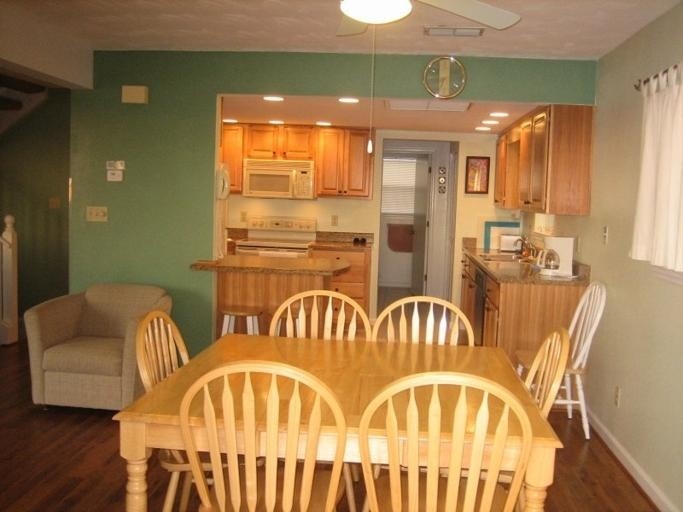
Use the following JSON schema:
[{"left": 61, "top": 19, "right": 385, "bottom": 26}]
[{"left": 335, "top": 0, "right": 522, "bottom": 37}]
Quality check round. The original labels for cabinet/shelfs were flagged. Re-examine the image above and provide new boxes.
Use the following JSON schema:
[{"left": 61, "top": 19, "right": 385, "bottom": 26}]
[
  {"left": 490, "top": 124, "right": 523, "bottom": 211},
  {"left": 221, "top": 125, "right": 243, "bottom": 194},
  {"left": 312, "top": 248, "right": 372, "bottom": 339},
  {"left": 519, "top": 105, "right": 590, "bottom": 216},
  {"left": 316, "top": 126, "right": 374, "bottom": 201},
  {"left": 458, "top": 255, "right": 486, "bottom": 343},
  {"left": 481, "top": 277, "right": 592, "bottom": 411},
  {"left": 246, "top": 124, "right": 314, "bottom": 159}
]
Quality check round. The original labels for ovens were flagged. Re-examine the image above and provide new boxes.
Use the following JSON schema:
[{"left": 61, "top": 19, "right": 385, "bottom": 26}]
[{"left": 234, "top": 246, "right": 311, "bottom": 259}]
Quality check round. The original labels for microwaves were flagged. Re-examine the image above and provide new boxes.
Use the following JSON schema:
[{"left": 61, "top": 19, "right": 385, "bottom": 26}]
[{"left": 242, "top": 158, "right": 315, "bottom": 199}]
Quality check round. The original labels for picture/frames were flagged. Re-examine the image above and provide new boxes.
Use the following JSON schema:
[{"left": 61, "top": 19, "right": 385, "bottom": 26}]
[{"left": 465, "top": 155, "right": 489, "bottom": 195}]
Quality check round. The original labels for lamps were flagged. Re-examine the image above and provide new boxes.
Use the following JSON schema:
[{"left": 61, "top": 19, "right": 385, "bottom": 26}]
[{"left": 339, "top": 1, "right": 413, "bottom": 26}]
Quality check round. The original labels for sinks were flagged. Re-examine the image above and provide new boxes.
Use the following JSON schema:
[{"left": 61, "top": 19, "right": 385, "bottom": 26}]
[{"left": 478, "top": 254, "right": 524, "bottom": 262}]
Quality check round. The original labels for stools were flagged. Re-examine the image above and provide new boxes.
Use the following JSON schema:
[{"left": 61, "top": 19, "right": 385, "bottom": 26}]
[
  {"left": 268, "top": 304, "right": 311, "bottom": 340},
  {"left": 218, "top": 306, "right": 261, "bottom": 338}
]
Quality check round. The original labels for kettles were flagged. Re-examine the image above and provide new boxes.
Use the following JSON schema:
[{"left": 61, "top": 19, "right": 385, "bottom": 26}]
[{"left": 536, "top": 248, "right": 559, "bottom": 270}]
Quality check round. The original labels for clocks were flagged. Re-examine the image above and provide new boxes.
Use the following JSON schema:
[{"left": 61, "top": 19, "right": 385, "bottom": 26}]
[{"left": 422, "top": 55, "right": 467, "bottom": 99}]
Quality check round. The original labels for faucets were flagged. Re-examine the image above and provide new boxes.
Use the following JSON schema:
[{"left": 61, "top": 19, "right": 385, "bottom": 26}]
[{"left": 513, "top": 238, "right": 528, "bottom": 255}]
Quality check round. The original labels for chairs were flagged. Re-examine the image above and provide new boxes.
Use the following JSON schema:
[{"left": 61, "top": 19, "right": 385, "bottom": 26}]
[
  {"left": 515, "top": 280, "right": 606, "bottom": 441},
  {"left": 20, "top": 280, "right": 174, "bottom": 415},
  {"left": 359, "top": 371, "right": 533, "bottom": 512},
  {"left": 440, "top": 328, "right": 569, "bottom": 512},
  {"left": 179, "top": 360, "right": 346, "bottom": 512},
  {"left": 267, "top": 290, "right": 371, "bottom": 484},
  {"left": 371, "top": 294, "right": 475, "bottom": 485},
  {"left": 133, "top": 308, "right": 265, "bottom": 512}
]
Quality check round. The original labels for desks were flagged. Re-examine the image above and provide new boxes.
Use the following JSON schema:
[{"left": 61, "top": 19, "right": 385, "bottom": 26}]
[
  {"left": 112, "top": 335, "right": 563, "bottom": 512},
  {"left": 190, "top": 253, "right": 351, "bottom": 336}
]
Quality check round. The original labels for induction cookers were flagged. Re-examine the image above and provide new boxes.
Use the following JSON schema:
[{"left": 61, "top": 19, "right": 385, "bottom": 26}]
[{"left": 235, "top": 237, "right": 313, "bottom": 248}]
